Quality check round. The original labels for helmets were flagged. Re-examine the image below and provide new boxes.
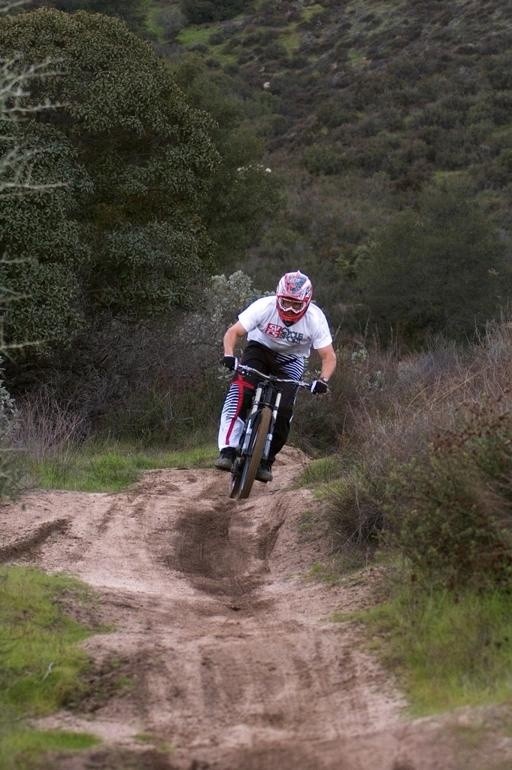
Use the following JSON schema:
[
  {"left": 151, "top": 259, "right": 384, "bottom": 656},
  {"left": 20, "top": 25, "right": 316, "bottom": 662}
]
[{"left": 275, "top": 269, "right": 312, "bottom": 327}]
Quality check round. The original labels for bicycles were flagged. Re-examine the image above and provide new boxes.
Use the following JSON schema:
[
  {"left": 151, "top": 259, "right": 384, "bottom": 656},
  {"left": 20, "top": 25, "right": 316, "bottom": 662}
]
[{"left": 222, "top": 357, "right": 327, "bottom": 504}]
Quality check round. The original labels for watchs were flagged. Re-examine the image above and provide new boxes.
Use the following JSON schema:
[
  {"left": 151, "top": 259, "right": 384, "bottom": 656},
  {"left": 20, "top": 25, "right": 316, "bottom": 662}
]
[{"left": 319, "top": 376, "right": 329, "bottom": 384}]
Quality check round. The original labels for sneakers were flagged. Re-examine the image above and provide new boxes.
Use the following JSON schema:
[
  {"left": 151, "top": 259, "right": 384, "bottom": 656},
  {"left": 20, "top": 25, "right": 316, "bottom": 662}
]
[
  {"left": 256, "top": 458, "right": 273, "bottom": 481},
  {"left": 215, "top": 447, "right": 236, "bottom": 470}
]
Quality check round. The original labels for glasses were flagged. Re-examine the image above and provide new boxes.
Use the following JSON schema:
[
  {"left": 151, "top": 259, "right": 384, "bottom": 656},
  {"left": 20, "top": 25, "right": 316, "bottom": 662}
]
[{"left": 277, "top": 296, "right": 311, "bottom": 314}]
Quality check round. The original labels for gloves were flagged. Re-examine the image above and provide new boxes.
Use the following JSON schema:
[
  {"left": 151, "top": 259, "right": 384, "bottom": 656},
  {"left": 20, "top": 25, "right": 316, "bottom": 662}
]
[
  {"left": 310, "top": 378, "right": 329, "bottom": 395},
  {"left": 218, "top": 356, "right": 238, "bottom": 381}
]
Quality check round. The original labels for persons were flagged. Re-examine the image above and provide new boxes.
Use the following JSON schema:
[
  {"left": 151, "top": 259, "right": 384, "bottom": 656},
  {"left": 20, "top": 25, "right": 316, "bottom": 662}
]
[{"left": 213, "top": 268, "right": 338, "bottom": 481}]
